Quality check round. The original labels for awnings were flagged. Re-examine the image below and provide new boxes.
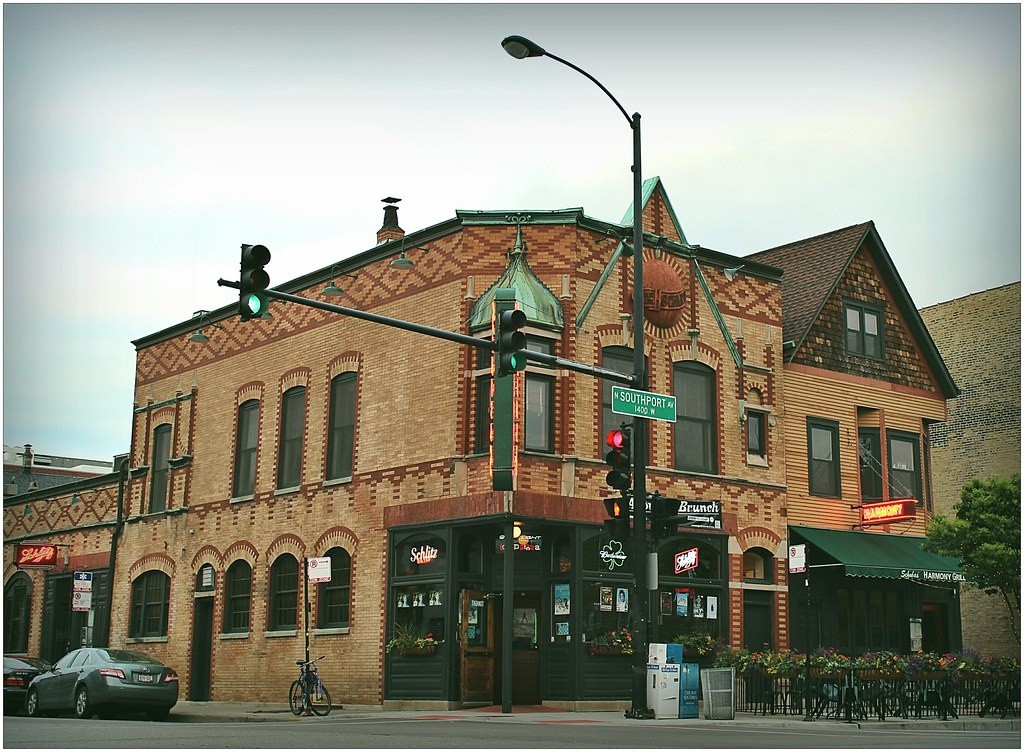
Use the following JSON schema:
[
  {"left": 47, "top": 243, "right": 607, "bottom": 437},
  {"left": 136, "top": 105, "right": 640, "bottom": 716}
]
[{"left": 788, "top": 526, "right": 984, "bottom": 583}]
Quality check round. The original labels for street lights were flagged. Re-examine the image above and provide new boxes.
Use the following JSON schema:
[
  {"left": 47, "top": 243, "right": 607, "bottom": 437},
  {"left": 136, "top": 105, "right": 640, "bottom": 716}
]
[{"left": 502, "top": 32, "right": 659, "bottom": 720}]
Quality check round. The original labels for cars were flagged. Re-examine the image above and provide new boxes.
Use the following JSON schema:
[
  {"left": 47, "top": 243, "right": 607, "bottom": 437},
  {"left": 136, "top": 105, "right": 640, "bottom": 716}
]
[
  {"left": 3, "top": 653, "right": 52, "bottom": 716},
  {"left": 24, "top": 647, "right": 178, "bottom": 720}
]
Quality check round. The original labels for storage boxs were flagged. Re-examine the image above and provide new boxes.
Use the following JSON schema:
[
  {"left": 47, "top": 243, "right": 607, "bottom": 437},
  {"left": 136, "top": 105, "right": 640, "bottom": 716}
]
[{"left": 649, "top": 643, "right": 683, "bottom": 663}]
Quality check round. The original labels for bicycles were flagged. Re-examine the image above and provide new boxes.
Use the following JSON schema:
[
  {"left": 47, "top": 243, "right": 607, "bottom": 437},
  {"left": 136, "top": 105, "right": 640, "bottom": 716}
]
[{"left": 288, "top": 655, "right": 332, "bottom": 717}]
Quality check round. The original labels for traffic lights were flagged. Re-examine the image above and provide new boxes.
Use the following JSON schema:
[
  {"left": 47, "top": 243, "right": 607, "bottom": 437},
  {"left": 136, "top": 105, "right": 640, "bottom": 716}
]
[
  {"left": 497, "top": 310, "right": 529, "bottom": 377},
  {"left": 603, "top": 429, "right": 631, "bottom": 491},
  {"left": 601, "top": 496, "right": 630, "bottom": 543},
  {"left": 240, "top": 244, "right": 271, "bottom": 319}
]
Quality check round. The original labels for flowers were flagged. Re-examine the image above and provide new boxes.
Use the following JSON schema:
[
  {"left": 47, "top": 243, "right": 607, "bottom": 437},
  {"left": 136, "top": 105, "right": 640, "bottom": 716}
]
[
  {"left": 710, "top": 643, "right": 1021, "bottom": 679},
  {"left": 673, "top": 632, "right": 722, "bottom": 655},
  {"left": 590, "top": 628, "right": 634, "bottom": 657},
  {"left": 386, "top": 620, "right": 445, "bottom": 654}
]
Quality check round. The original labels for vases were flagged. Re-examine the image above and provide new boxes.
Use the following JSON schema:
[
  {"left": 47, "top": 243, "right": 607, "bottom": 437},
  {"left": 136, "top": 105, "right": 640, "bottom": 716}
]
[
  {"left": 685, "top": 649, "right": 714, "bottom": 657},
  {"left": 597, "top": 646, "right": 625, "bottom": 655},
  {"left": 737, "top": 669, "right": 1020, "bottom": 681},
  {"left": 396, "top": 645, "right": 436, "bottom": 655}
]
